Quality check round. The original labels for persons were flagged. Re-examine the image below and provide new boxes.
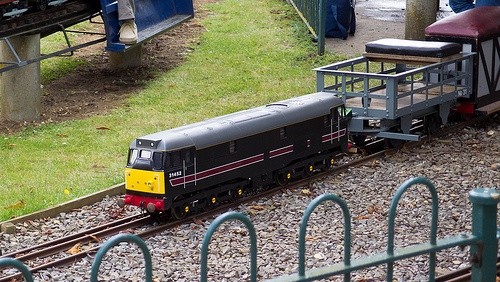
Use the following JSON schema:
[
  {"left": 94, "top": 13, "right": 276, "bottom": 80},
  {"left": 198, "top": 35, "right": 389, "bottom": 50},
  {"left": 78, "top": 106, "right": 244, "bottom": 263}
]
[
  {"left": 118, "top": 0, "right": 138, "bottom": 45},
  {"left": 449, "top": 0, "right": 500, "bottom": 14}
]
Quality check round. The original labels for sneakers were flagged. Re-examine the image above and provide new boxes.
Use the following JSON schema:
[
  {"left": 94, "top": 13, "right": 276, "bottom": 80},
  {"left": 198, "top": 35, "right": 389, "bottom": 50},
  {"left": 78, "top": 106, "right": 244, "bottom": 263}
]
[{"left": 119, "top": 22, "right": 139, "bottom": 45}]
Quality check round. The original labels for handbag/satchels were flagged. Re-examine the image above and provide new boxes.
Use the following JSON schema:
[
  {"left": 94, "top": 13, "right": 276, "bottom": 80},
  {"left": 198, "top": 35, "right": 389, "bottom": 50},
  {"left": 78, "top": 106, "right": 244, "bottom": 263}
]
[{"left": 325, "top": 0, "right": 356, "bottom": 39}]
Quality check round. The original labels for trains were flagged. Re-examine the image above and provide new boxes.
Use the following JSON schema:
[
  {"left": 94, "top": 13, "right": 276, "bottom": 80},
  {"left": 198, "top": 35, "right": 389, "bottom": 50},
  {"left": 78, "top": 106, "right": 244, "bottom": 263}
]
[{"left": 124, "top": 6, "right": 500, "bottom": 223}]
